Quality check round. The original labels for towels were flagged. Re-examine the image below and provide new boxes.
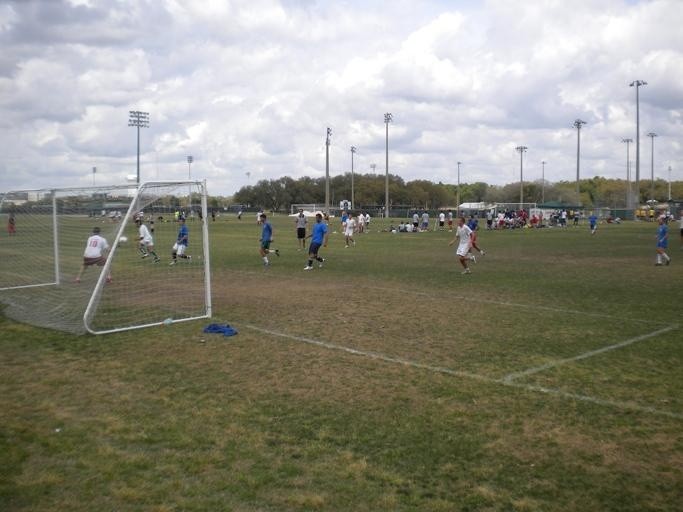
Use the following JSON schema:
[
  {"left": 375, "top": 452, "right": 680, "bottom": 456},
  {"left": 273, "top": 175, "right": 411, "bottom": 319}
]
[{"left": 204, "top": 321, "right": 237, "bottom": 336}]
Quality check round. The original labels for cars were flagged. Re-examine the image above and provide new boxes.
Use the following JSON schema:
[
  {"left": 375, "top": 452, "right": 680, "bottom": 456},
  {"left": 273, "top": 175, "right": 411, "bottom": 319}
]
[
  {"left": 634, "top": 205, "right": 655, "bottom": 218},
  {"left": 645, "top": 199, "right": 659, "bottom": 205}
]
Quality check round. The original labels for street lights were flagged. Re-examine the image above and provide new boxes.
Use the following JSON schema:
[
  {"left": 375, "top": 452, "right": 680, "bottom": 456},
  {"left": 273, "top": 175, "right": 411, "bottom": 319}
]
[
  {"left": 90, "top": 165, "right": 97, "bottom": 187},
  {"left": 455, "top": 161, "right": 463, "bottom": 218},
  {"left": 629, "top": 77, "right": 647, "bottom": 204},
  {"left": 647, "top": 133, "right": 659, "bottom": 207},
  {"left": 667, "top": 164, "right": 673, "bottom": 203},
  {"left": 568, "top": 118, "right": 587, "bottom": 205},
  {"left": 621, "top": 137, "right": 632, "bottom": 208},
  {"left": 126, "top": 110, "right": 150, "bottom": 191},
  {"left": 321, "top": 109, "right": 394, "bottom": 222},
  {"left": 539, "top": 160, "right": 547, "bottom": 205},
  {"left": 185, "top": 156, "right": 194, "bottom": 210},
  {"left": 514, "top": 145, "right": 528, "bottom": 207}
]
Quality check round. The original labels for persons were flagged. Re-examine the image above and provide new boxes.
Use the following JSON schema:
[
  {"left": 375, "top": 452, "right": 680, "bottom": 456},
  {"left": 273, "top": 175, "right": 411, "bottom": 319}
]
[
  {"left": 76, "top": 226, "right": 112, "bottom": 285},
  {"left": 9, "top": 211, "right": 17, "bottom": 236},
  {"left": 168, "top": 217, "right": 192, "bottom": 267},
  {"left": 133, "top": 218, "right": 160, "bottom": 265},
  {"left": 101, "top": 208, "right": 243, "bottom": 233}
]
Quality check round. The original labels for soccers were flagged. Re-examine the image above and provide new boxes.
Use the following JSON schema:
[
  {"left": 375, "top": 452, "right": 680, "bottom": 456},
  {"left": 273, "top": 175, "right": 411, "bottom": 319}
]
[{"left": 119, "top": 236, "right": 127, "bottom": 242}]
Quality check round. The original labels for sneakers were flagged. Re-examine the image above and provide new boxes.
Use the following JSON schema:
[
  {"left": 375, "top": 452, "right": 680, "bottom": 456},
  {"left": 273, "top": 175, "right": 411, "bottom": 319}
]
[
  {"left": 471, "top": 256, "right": 476, "bottom": 264},
  {"left": 319, "top": 259, "right": 325, "bottom": 268},
  {"left": 276, "top": 250, "right": 280, "bottom": 256},
  {"left": 304, "top": 266, "right": 313, "bottom": 270},
  {"left": 461, "top": 270, "right": 471, "bottom": 275}
]
